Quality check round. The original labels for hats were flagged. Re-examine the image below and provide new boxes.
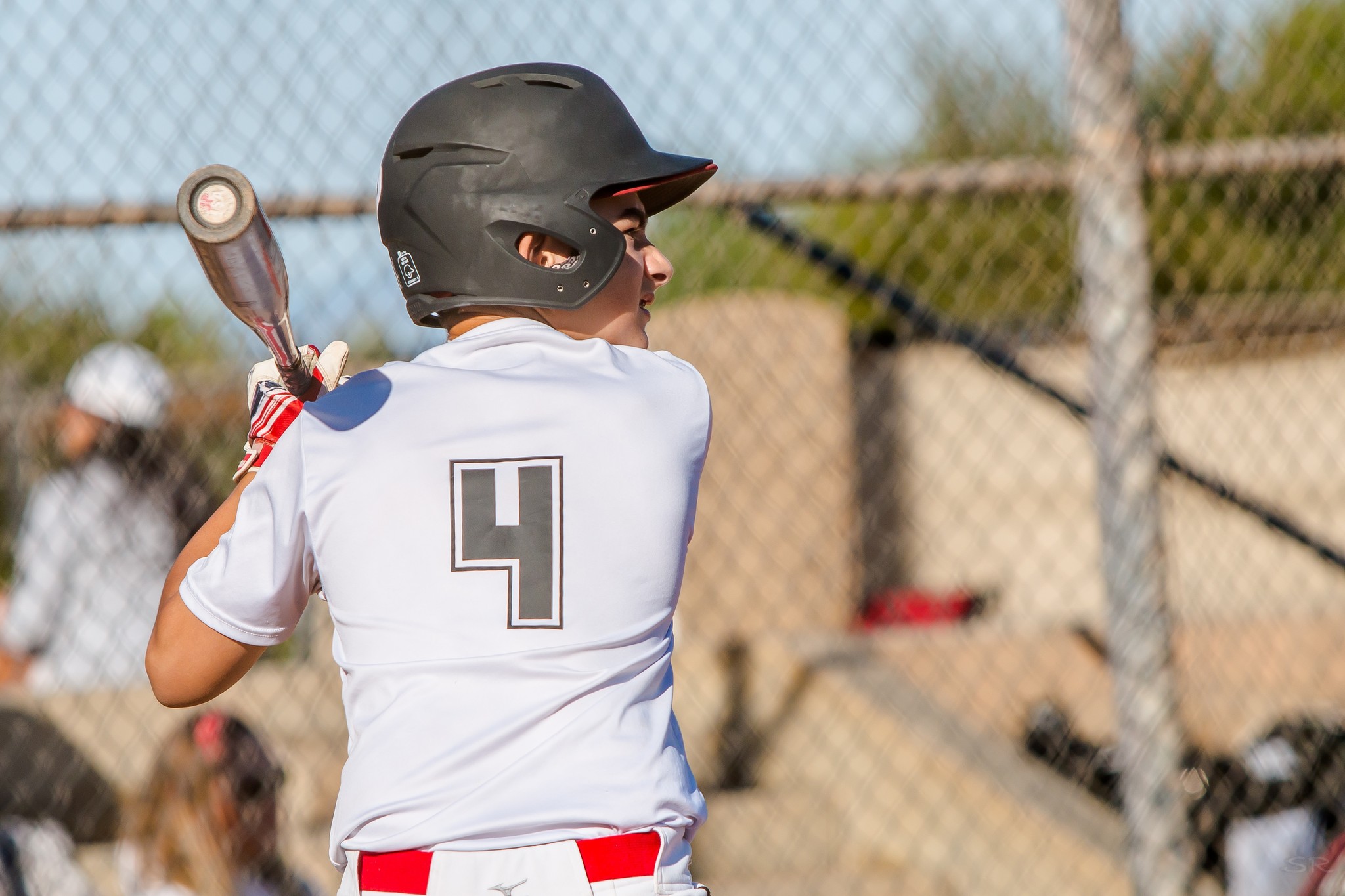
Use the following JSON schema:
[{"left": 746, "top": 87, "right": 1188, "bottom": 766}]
[
  {"left": 593, "top": 164, "right": 718, "bottom": 216},
  {"left": 65, "top": 342, "right": 171, "bottom": 428}
]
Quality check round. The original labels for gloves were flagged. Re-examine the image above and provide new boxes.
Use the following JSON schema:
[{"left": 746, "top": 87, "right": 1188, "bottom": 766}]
[{"left": 232, "top": 340, "right": 352, "bottom": 485}]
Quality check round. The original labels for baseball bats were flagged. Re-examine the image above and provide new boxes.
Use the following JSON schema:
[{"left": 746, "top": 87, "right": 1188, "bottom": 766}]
[{"left": 174, "top": 163, "right": 312, "bottom": 401}]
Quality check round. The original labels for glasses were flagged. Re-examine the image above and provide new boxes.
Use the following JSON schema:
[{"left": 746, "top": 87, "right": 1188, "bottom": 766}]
[{"left": 237, "top": 768, "right": 288, "bottom": 804}]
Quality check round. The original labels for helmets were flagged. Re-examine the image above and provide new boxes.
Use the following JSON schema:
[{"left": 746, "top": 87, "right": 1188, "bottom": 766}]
[{"left": 376, "top": 63, "right": 718, "bottom": 328}]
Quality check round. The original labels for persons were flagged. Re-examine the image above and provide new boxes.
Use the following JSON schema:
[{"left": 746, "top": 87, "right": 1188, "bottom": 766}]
[
  {"left": 0, "top": 335, "right": 306, "bottom": 896},
  {"left": 143, "top": 62, "right": 720, "bottom": 896}
]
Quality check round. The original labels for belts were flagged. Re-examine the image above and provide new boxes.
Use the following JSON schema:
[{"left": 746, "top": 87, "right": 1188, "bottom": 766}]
[{"left": 358, "top": 832, "right": 660, "bottom": 895}]
